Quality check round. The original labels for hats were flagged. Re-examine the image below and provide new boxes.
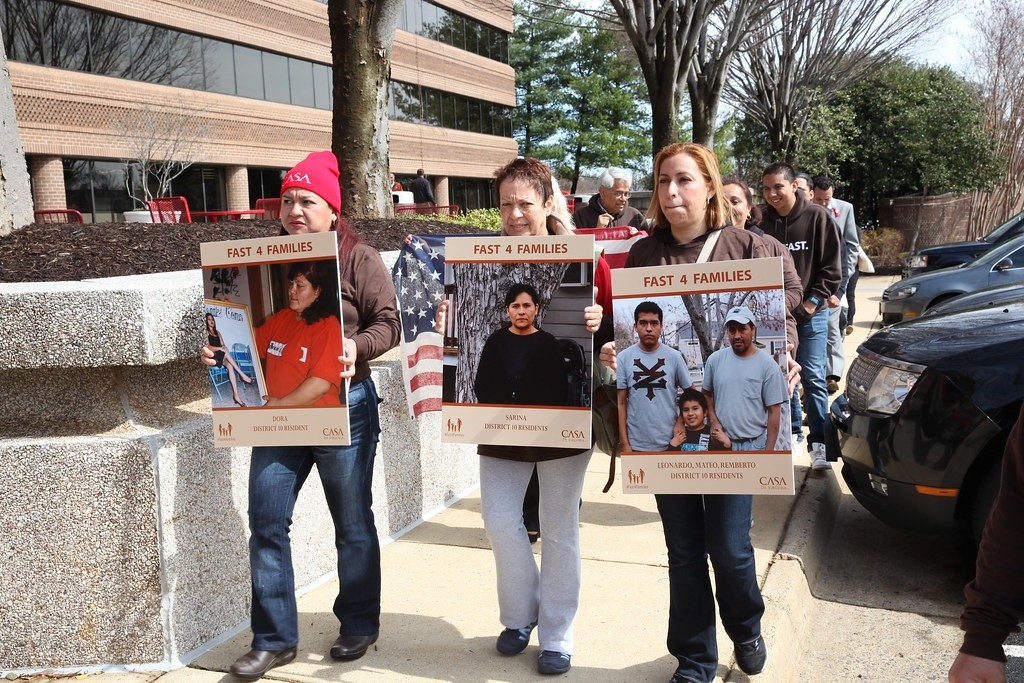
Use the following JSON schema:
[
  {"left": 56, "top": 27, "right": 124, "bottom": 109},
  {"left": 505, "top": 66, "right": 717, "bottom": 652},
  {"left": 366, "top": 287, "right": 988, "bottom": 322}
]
[
  {"left": 724, "top": 306, "right": 756, "bottom": 327},
  {"left": 280, "top": 151, "right": 341, "bottom": 214}
]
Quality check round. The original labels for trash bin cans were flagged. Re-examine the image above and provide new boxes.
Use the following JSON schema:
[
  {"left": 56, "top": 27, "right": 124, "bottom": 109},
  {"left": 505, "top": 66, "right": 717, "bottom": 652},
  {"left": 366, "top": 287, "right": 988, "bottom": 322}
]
[
  {"left": 574, "top": 195, "right": 592, "bottom": 213},
  {"left": 392, "top": 191, "right": 415, "bottom": 215}
]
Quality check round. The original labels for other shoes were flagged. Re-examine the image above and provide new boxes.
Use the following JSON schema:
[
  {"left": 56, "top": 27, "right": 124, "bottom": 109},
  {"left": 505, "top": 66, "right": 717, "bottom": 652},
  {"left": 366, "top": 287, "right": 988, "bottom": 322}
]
[{"left": 846, "top": 325, "right": 853, "bottom": 334}]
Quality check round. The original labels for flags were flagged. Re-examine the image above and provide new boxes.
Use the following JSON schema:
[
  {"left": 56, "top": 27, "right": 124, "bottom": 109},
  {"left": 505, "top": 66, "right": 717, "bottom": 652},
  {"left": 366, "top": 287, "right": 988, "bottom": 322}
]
[{"left": 392, "top": 231, "right": 444, "bottom": 414}]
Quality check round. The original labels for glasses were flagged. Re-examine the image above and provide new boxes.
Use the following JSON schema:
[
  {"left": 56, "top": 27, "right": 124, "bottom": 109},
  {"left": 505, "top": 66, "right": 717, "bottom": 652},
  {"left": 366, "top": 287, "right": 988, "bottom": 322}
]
[{"left": 606, "top": 190, "right": 631, "bottom": 199}]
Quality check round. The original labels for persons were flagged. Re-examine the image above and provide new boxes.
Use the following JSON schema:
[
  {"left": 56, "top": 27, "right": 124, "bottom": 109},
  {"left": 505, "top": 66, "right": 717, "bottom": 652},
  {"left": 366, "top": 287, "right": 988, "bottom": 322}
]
[
  {"left": 204, "top": 309, "right": 255, "bottom": 407},
  {"left": 390, "top": 171, "right": 403, "bottom": 191},
  {"left": 795, "top": 165, "right": 876, "bottom": 394},
  {"left": 201, "top": 150, "right": 402, "bottom": 682},
  {"left": 474, "top": 284, "right": 570, "bottom": 406},
  {"left": 600, "top": 142, "right": 801, "bottom": 683},
  {"left": 704, "top": 306, "right": 789, "bottom": 451},
  {"left": 574, "top": 162, "right": 648, "bottom": 231},
  {"left": 754, "top": 165, "right": 844, "bottom": 471},
  {"left": 669, "top": 390, "right": 732, "bottom": 452},
  {"left": 616, "top": 301, "right": 693, "bottom": 454},
  {"left": 946, "top": 411, "right": 1024, "bottom": 683},
  {"left": 436, "top": 158, "right": 605, "bottom": 677},
  {"left": 252, "top": 262, "right": 343, "bottom": 408},
  {"left": 719, "top": 175, "right": 803, "bottom": 355},
  {"left": 409, "top": 169, "right": 438, "bottom": 207}
]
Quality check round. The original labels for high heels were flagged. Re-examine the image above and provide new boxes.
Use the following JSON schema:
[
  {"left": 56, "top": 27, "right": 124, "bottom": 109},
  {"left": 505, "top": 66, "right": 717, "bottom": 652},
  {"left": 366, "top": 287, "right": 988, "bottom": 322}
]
[
  {"left": 239, "top": 374, "right": 257, "bottom": 385},
  {"left": 233, "top": 397, "right": 246, "bottom": 407}
]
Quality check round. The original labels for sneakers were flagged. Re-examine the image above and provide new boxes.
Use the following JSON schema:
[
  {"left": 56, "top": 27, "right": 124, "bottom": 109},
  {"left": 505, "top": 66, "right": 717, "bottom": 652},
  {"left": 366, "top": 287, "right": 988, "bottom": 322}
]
[
  {"left": 809, "top": 443, "right": 832, "bottom": 470},
  {"left": 497, "top": 617, "right": 538, "bottom": 654},
  {"left": 669, "top": 673, "right": 695, "bottom": 683},
  {"left": 735, "top": 633, "right": 767, "bottom": 675},
  {"left": 538, "top": 650, "right": 570, "bottom": 673}
]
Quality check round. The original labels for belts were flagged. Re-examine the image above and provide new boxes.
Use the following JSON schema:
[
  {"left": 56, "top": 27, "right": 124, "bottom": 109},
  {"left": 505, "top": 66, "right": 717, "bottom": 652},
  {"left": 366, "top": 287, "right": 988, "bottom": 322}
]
[{"left": 729, "top": 438, "right": 756, "bottom": 443}]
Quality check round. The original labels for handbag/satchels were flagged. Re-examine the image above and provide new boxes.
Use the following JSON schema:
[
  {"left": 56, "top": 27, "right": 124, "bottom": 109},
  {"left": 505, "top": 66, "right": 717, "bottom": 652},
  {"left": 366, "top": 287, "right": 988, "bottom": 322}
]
[{"left": 593, "top": 355, "right": 625, "bottom": 493}]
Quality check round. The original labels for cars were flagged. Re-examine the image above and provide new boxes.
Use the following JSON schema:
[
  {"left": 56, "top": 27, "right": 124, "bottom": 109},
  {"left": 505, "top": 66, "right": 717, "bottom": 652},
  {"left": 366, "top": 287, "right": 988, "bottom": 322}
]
[{"left": 830, "top": 211, "right": 1024, "bottom": 573}]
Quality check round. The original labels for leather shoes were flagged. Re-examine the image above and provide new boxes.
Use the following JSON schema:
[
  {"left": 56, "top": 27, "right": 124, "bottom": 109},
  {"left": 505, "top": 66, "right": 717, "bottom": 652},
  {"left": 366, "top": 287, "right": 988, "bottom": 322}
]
[
  {"left": 827, "top": 378, "right": 839, "bottom": 392},
  {"left": 330, "top": 629, "right": 379, "bottom": 658},
  {"left": 230, "top": 646, "right": 297, "bottom": 679}
]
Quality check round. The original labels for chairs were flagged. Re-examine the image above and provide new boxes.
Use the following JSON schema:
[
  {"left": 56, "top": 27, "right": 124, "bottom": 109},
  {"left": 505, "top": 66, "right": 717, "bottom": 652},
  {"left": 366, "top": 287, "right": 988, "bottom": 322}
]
[{"left": 209, "top": 342, "right": 257, "bottom": 401}]
